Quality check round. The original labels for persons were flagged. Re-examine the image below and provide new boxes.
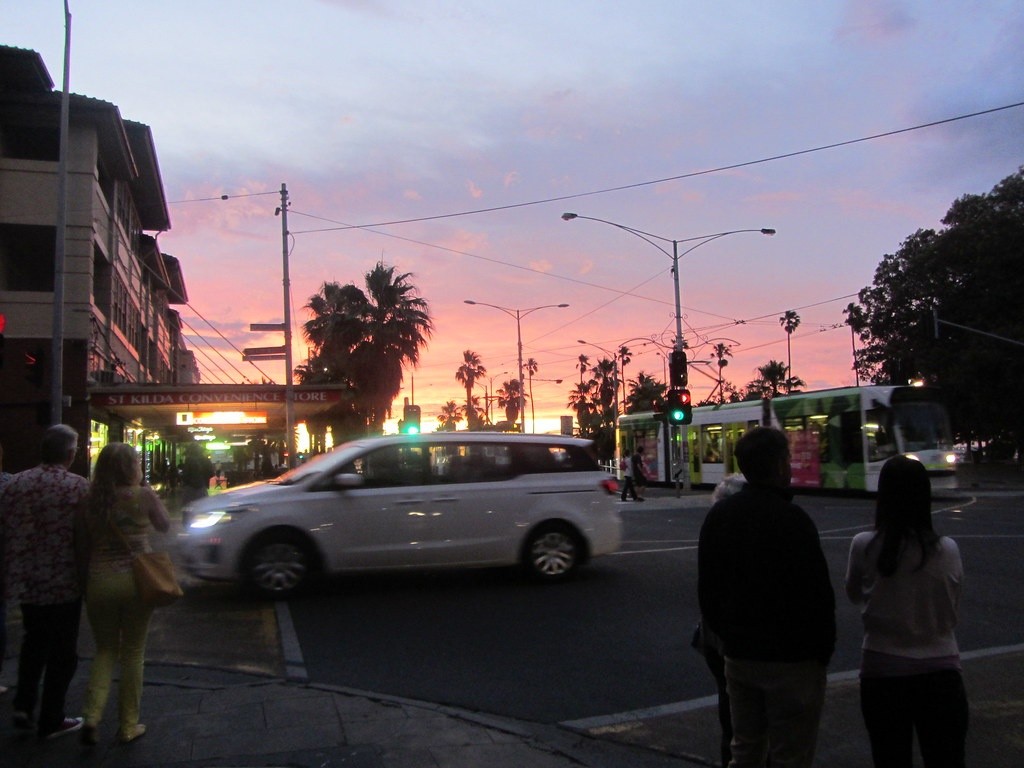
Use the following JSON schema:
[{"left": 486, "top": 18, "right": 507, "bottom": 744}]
[
  {"left": 621, "top": 450, "right": 645, "bottom": 501},
  {"left": 695, "top": 427, "right": 837, "bottom": 768},
  {"left": 692, "top": 473, "right": 746, "bottom": 768},
  {"left": 844, "top": 455, "right": 969, "bottom": 768},
  {"left": 630, "top": 447, "right": 648, "bottom": 499},
  {"left": 72, "top": 441, "right": 170, "bottom": 742},
  {"left": 0, "top": 424, "right": 92, "bottom": 738}
]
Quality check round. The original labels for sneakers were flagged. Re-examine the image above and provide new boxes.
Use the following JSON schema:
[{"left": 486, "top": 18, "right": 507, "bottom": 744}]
[
  {"left": 12, "top": 711, "right": 36, "bottom": 736},
  {"left": 45, "top": 716, "right": 85, "bottom": 739}
]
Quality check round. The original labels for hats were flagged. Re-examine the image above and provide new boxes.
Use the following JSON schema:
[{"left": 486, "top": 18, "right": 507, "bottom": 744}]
[{"left": 712, "top": 472, "right": 747, "bottom": 506}]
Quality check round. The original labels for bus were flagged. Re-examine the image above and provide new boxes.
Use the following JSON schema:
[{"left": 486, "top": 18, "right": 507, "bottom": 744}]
[{"left": 614, "top": 383, "right": 961, "bottom": 499}]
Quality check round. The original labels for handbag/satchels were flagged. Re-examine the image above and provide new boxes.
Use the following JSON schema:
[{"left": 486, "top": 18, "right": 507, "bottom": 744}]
[
  {"left": 620, "top": 458, "right": 626, "bottom": 470},
  {"left": 130, "top": 552, "right": 184, "bottom": 606}
]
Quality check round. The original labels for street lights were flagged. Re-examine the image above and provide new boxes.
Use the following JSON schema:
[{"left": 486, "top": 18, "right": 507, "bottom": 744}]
[
  {"left": 577, "top": 340, "right": 618, "bottom": 420},
  {"left": 463, "top": 300, "right": 569, "bottom": 432},
  {"left": 562, "top": 212, "right": 776, "bottom": 495},
  {"left": 474, "top": 370, "right": 509, "bottom": 427}
]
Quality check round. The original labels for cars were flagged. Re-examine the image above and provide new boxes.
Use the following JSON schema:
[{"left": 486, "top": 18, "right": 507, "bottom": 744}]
[
  {"left": 169, "top": 430, "right": 625, "bottom": 606},
  {"left": 435, "top": 442, "right": 573, "bottom": 478}
]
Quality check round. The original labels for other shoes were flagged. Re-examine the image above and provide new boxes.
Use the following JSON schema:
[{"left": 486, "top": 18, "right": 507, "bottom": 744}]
[
  {"left": 80, "top": 718, "right": 99, "bottom": 744},
  {"left": 120, "top": 724, "right": 145, "bottom": 742}
]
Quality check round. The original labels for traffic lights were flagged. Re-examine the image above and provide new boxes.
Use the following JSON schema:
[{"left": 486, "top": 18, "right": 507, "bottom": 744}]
[
  {"left": 671, "top": 351, "right": 688, "bottom": 386},
  {"left": 403, "top": 404, "right": 421, "bottom": 434},
  {"left": 667, "top": 388, "right": 692, "bottom": 427}
]
